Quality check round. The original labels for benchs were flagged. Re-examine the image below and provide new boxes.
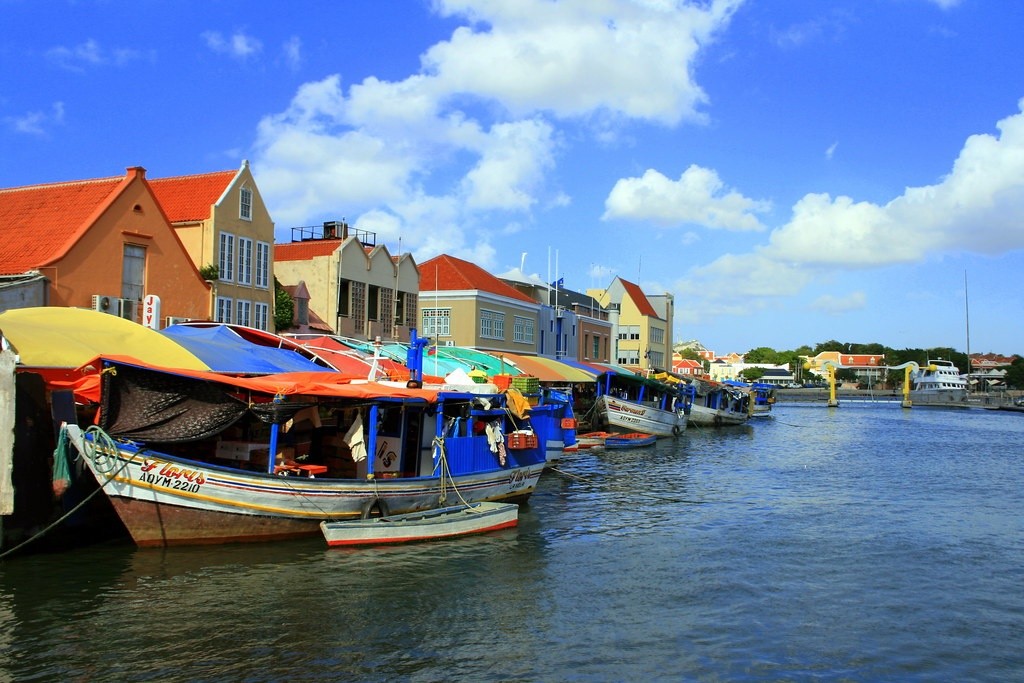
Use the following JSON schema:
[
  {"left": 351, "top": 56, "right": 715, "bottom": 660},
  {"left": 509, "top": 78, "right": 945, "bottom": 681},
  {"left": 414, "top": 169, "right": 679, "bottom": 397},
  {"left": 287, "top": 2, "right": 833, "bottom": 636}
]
[{"left": 295, "top": 462, "right": 328, "bottom": 477}]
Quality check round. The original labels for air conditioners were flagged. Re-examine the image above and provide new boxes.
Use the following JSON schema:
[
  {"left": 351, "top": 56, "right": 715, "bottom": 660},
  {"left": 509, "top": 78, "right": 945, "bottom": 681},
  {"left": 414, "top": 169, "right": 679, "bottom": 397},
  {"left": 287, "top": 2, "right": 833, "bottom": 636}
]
[
  {"left": 165, "top": 317, "right": 200, "bottom": 328},
  {"left": 118, "top": 299, "right": 138, "bottom": 323},
  {"left": 91, "top": 294, "right": 119, "bottom": 318},
  {"left": 446, "top": 341, "right": 455, "bottom": 346}
]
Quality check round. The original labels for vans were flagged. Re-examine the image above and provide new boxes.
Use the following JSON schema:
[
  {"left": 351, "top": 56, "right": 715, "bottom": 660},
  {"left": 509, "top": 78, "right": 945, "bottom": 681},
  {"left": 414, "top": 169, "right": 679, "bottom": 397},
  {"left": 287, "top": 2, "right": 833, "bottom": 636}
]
[{"left": 787, "top": 383, "right": 801, "bottom": 388}]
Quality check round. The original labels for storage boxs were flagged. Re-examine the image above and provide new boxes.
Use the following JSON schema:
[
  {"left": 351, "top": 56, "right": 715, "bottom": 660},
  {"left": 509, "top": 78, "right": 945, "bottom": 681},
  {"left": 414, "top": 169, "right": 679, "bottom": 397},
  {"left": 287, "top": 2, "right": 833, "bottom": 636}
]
[
  {"left": 561, "top": 418, "right": 578, "bottom": 429},
  {"left": 215, "top": 439, "right": 271, "bottom": 459},
  {"left": 472, "top": 376, "right": 488, "bottom": 383},
  {"left": 493, "top": 375, "right": 539, "bottom": 405},
  {"left": 508, "top": 433, "right": 538, "bottom": 449},
  {"left": 322, "top": 430, "right": 358, "bottom": 478},
  {"left": 649, "top": 372, "right": 681, "bottom": 384}
]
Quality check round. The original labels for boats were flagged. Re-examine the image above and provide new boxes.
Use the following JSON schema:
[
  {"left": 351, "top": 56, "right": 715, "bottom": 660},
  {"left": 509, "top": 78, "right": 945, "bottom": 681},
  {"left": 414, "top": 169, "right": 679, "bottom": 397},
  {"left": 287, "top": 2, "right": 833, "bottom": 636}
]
[
  {"left": 596, "top": 372, "right": 697, "bottom": 437},
  {"left": 690, "top": 376, "right": 751, "bottom": 426},
  {"left": 910, "top": 357, "right": 968, "bottom": 404},
  {"left": 66, "top": 397, "right": 548, "bottom": 552},
  {"left": 750, "top": 383, "right": 775, "bottom": 417},
  {"left": 575, "top": 432, "right": 620, "bottom": 448},
  {"left": 604, "top": 433, "right": 658, "bottom": 450}
]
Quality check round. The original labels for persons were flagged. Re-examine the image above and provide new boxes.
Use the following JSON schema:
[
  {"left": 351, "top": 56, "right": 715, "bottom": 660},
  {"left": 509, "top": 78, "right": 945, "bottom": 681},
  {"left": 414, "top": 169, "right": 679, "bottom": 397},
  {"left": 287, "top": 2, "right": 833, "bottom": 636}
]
[
  {"left": 352, "top": 409, "right": 358, "bottom": 424},
  {"left": 335, "top": 412, "right": 347, "bottom": 436},
  {"left": 722, "top": 396, "right": 750, "bottom": 412},
  {"left": 542, "top": 381, "right": 596, "bottom": 424}
]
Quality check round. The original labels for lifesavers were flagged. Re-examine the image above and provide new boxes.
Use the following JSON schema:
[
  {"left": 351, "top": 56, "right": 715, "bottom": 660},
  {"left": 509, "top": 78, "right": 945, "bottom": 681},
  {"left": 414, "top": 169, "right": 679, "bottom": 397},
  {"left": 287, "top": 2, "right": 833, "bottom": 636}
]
[
  {"left": 671, "top": 425, "right": 680, "bottom": 437},
  {"left": 362, "top": 499, "right": 389, "bottom": 519},
  {"left": 714, "top": 415, "right": 722, "bottom": 425}
]
